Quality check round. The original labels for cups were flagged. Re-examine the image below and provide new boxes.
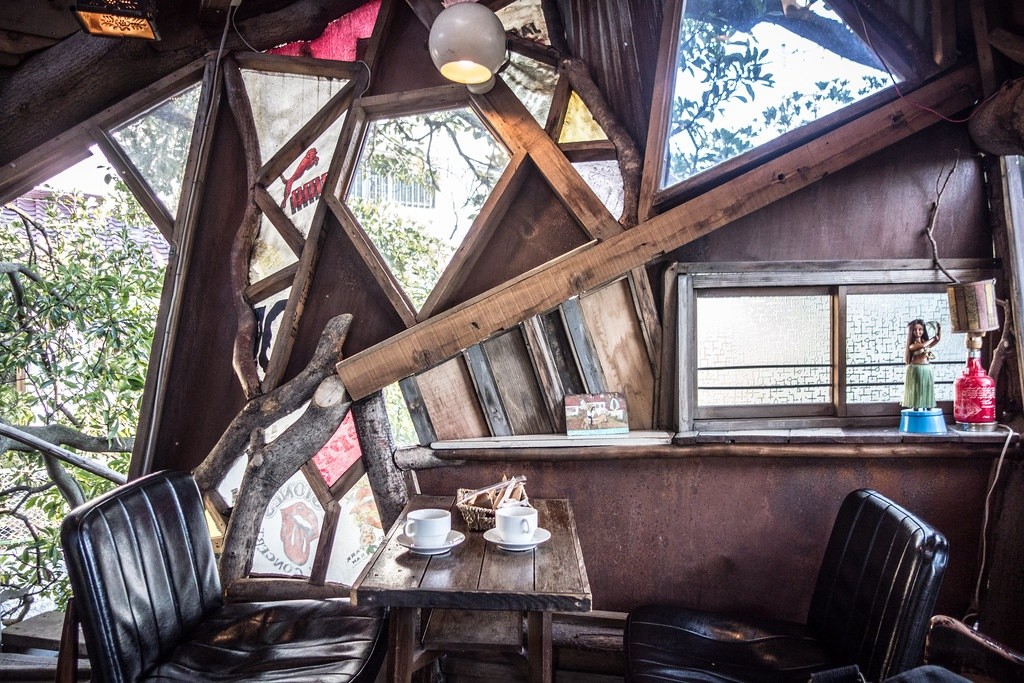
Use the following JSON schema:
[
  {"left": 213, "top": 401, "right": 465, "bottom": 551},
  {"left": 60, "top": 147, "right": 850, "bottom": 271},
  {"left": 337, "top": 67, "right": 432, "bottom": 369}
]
[
  {"left": 403, "top": 509, "right": 452, "bottom": 547},
  {"left": 495, "top": 507, "right": 539, "bottom": 543}
]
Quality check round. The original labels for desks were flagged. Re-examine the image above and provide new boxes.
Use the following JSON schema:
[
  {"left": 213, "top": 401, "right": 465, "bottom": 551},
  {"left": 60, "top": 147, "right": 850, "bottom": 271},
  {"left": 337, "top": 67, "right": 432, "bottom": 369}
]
[{"left": 351, "top": 494, "right": 593, "bottom": 683}]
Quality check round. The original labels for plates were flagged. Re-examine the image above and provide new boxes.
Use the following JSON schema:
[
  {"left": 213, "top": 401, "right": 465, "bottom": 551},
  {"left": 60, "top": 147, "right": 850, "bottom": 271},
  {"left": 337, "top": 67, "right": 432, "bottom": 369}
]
[
  {"left": 396, "top": 529, "right": 466, "bottom": 554},
  {"left": 483, "top": 527, "right": 551, "bottom": 552}
]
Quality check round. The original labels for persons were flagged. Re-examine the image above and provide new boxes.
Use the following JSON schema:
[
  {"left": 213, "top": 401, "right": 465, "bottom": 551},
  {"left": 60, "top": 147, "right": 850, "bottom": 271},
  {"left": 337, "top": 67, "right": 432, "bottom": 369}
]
[{"left": 900, "top": 318, "right": 943, "bottom": 411}]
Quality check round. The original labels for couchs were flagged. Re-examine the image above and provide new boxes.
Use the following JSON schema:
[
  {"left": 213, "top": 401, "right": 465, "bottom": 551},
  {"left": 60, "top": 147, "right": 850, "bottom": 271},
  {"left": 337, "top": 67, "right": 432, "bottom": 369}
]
[
  {"left": 619, "top": 485, "right": 950, "bottom": 682},
  {"left": 59, "top": 467, "right": 395, "bottom": 681}
]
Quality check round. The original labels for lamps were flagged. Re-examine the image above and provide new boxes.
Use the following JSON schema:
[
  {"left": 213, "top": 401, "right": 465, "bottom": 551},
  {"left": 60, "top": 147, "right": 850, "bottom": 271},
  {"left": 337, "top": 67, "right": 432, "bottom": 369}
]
[
  {"left": 70, "top": 2, "right": 163, "bottom": 46},
  {"left": 428, "top": 0, "right": 506, "bottom": 87}
]
